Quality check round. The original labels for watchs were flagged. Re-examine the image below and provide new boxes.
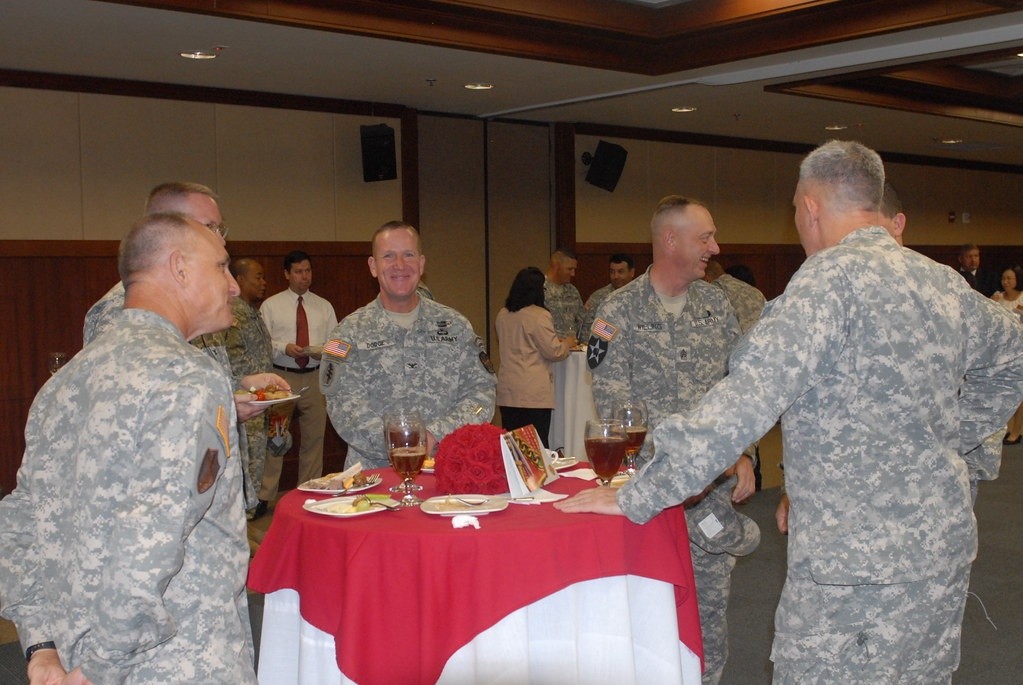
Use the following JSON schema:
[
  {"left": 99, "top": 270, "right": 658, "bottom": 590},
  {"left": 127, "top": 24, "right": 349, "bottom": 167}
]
[{"left": 26, "top": 641, "right": 56, "bottom": 661}]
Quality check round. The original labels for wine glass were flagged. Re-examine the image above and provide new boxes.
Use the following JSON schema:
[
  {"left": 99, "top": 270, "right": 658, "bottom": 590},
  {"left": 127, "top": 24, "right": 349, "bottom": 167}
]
[
  {"left": 613, "top": 399, "right": 649, "bottom": 479},
  {"left": 382, "top": 411, "right": 423, "bottom": 492},
  {"left": 584, "top": 419, "right": 628, "bottom": 487},
  {"left": 388, "top": 418, "right": 428, "bottom": 507}
]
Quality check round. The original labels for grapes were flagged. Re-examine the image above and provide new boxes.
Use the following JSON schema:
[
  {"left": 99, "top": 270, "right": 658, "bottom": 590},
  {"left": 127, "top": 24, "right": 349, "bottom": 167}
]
[{"left": 357, "top": 500, "right": 369, "bottom": 512}]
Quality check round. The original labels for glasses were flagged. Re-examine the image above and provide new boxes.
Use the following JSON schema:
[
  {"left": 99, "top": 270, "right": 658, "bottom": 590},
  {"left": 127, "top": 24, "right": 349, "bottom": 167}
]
[{"left": 204, "top": 222, "right": 228, "bottom": 238}]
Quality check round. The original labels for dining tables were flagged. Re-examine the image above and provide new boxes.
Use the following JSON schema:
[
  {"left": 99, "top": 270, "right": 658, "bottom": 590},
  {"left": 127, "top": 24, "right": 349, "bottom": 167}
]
[
  {"left": 547, "top": 343, "right": 602, "bottom": 462},
  {"left": 251, "top": 456, "right": 705, "bottom": 685}
]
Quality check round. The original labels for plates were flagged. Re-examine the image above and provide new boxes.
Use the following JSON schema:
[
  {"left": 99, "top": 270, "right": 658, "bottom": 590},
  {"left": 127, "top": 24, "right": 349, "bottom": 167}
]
[
  {"left": 552, "top": 458, "right": 579, "bottom": 470},
  {"left": 303, "top": 495, "right": 401, "bottom": 518},
  {"left": 596, "top": 475, "right": 630, "bottom": 487},
  {"left": 420, "top": 494, "right": 510, "bottom": 517},
  {"left": 248, "top": 393, "right": 301, "bottom": 406},
  {"left": 420, "top": 468, "right": 435, "bottom": 473},
  {"left": 297, "top": 478, "right": 383, "bottom": 496}
]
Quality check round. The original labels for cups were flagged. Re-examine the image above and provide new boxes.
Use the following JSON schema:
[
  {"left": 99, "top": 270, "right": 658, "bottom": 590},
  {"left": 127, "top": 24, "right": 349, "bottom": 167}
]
[
  {"left": 565, "top": 320, "right": 576, "bottom": 337},
  {"left": 544, "top": 449, "right": 559, "bottom": 464},
  {"left": 48, "top": 352, "right": 66, "bottom": 375}
]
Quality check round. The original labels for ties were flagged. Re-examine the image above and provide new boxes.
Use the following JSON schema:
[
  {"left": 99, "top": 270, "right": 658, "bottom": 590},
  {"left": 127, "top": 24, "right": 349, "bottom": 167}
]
[{"left": 295, "top": 296, "right": 309, "bottom": 368}]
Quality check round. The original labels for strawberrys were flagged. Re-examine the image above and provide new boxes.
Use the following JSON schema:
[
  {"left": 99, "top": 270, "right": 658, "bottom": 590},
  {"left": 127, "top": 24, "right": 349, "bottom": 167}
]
[{"left": 249, "top": 389, "right": 265, "bottom": 401}]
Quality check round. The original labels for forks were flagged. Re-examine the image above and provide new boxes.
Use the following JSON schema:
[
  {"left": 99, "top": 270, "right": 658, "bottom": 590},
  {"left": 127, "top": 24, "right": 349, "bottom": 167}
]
[
  {"left": 356, "top": 494, "right": 401, "bottom": 511},
  {"left": 331, "top": 474, "right": 380, "bottom": 497},
  {"left": 417, "top": 497, "right": 489, "bottom": 506}
]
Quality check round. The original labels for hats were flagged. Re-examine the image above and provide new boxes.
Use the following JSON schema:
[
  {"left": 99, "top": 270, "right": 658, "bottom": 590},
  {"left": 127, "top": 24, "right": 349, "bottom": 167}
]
[{"left": 688, "top": 474, "right": 761, "bottom": 556}]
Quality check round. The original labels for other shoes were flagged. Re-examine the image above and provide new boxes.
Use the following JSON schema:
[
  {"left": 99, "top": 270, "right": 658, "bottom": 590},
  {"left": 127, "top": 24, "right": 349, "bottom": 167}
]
[
  {"left": 1003, "top": 432, "right": 1021, "bottom": 445},
  {"left": 254, "top": 499, "right": 269, "bottom": 519}
]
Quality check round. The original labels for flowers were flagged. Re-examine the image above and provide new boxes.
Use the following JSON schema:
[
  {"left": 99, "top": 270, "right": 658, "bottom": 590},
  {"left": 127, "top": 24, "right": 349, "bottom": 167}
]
[{"left": 432, "top": 421, "right": 510, "bottom": 494}]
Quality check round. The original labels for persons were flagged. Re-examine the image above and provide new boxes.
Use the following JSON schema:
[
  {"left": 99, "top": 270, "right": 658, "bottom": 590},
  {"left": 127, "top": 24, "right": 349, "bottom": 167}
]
[
  {"left": 319, "top": 221, "right": 498, "bottom": 473},
  {"left": 586, "top": 194, "right": 761, "bottom": 684},
  {"left": 82, "top": 182, "right": 435, "bottom": 520},
  {"left": 0, "top": 212, "right": 240, "bottom": 685},
  {"left": 494, "top": 140, "right": 1023, "bottom": 684}
]
